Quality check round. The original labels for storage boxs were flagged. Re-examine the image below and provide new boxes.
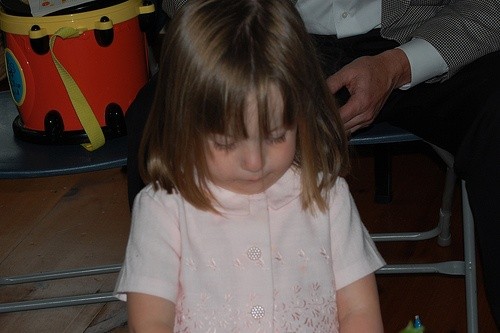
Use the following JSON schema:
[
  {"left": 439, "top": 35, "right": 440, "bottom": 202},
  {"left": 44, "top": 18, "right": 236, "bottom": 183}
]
[{"left": 0, "top": 0, "right": 157, "bottom": 140}]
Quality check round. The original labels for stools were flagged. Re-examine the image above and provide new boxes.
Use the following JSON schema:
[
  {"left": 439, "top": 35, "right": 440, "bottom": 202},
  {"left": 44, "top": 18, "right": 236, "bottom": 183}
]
[
  {"left": 332, "top": 120, "right": 478, "bottom": 333},
  {"left": 0, "top": 88, "right": 150, "bottom": 312}
]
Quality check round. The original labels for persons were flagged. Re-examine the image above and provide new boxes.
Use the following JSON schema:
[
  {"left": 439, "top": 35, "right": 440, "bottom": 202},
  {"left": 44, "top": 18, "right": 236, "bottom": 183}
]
[
  {"left": 288, "top": 0, "right": 496, "bottom": 202},
  {"left": 110, "top": 0, "right": 387, "bottom": 333}
]
